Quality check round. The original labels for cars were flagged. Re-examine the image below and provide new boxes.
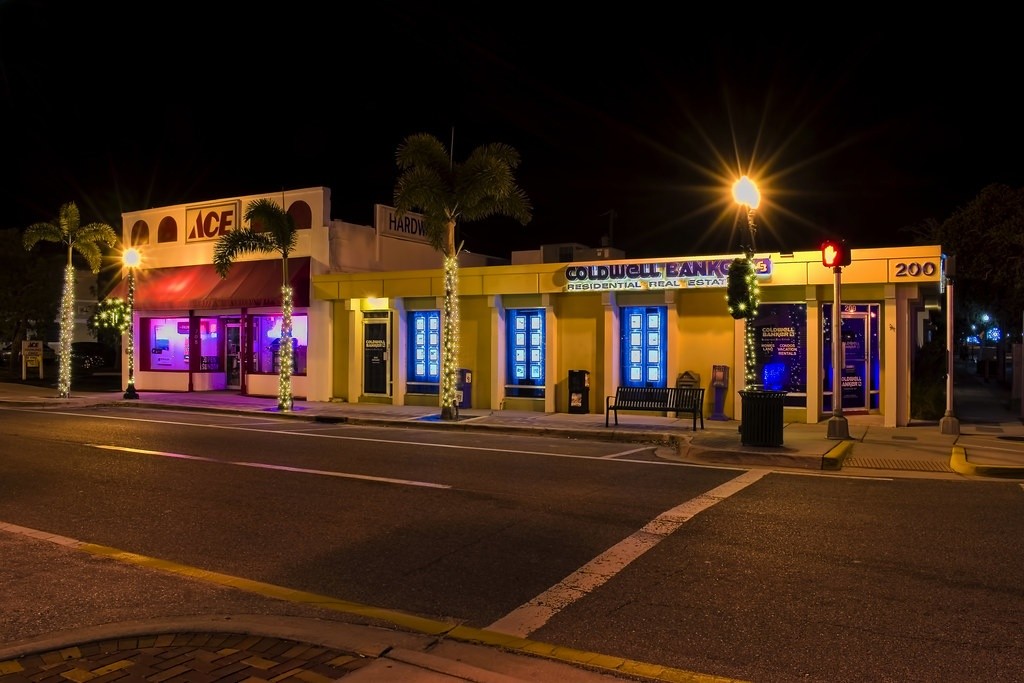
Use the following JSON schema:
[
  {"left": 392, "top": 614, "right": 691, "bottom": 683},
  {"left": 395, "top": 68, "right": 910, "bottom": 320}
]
[{"left": 0, "top": 343, "right": 56, "bottom": 369}]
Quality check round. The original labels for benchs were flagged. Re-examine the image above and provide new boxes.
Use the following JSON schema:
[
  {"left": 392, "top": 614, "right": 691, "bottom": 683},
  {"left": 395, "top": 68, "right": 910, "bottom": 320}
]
[{"left": 605, "top": 387, "right": 705, "bottom": 432}]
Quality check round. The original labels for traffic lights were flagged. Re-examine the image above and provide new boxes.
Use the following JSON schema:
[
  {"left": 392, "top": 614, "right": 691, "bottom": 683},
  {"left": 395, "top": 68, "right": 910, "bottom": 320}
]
[{"left": 822, "top": 242, "right": 851, "bottom": 268}]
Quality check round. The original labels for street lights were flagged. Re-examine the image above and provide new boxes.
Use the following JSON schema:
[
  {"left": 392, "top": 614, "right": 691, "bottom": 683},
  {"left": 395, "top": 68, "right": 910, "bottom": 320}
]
[
  {"left": 122, "top": 248, "right": 140, "bottom": 399},
  {"left": 971, "top": 324, "right": 976, "bottom": 359},
  {"left": 733, "top": 177, "right": 759, "bottom": 253},
  {"left": 983, "top": 314, "right": 989, "bottom": 354}
]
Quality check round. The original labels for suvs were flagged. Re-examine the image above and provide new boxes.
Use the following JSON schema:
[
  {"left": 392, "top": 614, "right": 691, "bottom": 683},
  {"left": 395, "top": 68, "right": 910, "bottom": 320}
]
[{"left": 71, "top": 342, "right": 115, "bottom": 369}]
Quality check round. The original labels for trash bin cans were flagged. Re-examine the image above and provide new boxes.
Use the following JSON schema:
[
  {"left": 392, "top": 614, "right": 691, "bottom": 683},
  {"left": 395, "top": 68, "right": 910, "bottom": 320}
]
[
  {"left": 738, "top": 390, "right": 788, "bottom": 446},
  {"left": 455, "top": 368, "right": 472, "bottom": 410},
  {"left": 569, "top": 369, "right": 590, "bottom": 414},
  {"left": 959, "top": 346, "right": 968, "bottom": 357}
]
